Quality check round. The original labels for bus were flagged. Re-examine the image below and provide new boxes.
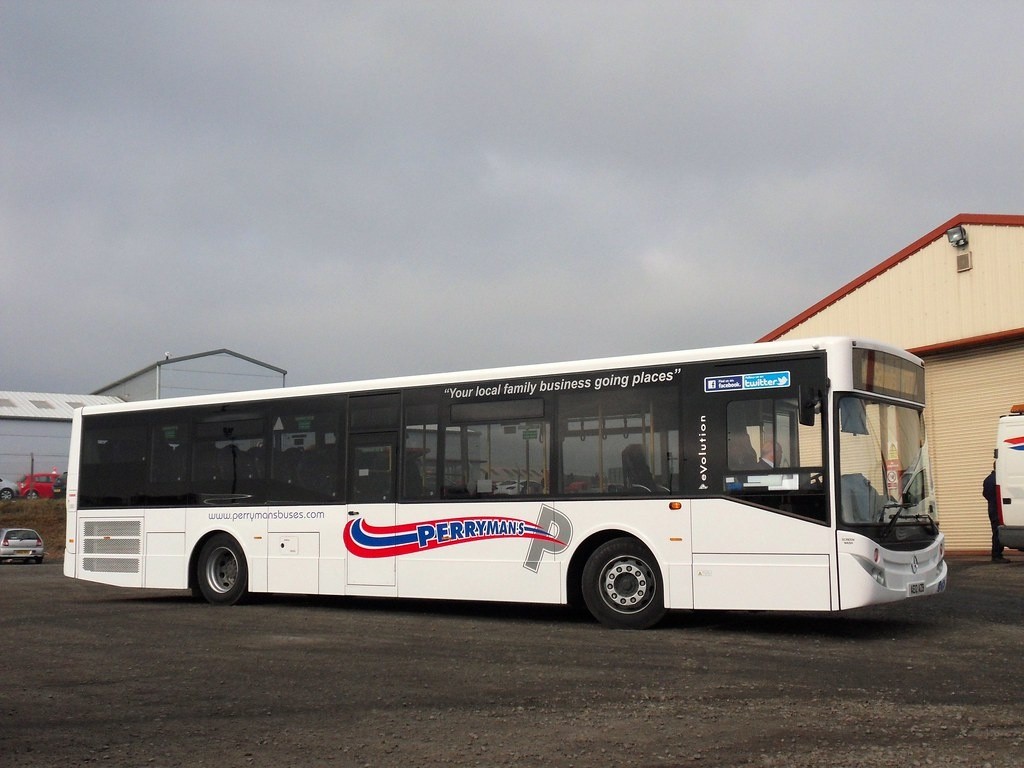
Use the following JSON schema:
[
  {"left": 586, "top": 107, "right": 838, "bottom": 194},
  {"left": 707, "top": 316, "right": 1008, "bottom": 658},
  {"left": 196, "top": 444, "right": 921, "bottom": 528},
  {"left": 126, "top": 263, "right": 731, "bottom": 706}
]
[{"left": 64, "top": 336, "right": 948, "bottom": 629}]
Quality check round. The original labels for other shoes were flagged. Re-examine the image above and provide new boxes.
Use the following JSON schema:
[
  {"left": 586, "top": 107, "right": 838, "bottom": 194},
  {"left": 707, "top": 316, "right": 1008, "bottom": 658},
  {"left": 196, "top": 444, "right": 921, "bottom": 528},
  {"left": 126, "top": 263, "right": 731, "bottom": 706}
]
[{"left": 991, "top": 556, "right": 1012, "bottom": 564}]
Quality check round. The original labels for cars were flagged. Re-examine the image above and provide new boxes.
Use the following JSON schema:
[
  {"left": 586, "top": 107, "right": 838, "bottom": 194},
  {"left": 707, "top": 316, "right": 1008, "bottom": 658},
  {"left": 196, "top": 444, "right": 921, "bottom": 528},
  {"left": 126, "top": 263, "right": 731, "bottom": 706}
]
[
  {"left": 15, "top": 473, "right": 61, "bottom": 500},
  {"left": 997, "top": 404, "right": 1023, "bottom": 549},
  {"left": 0, "top": 477, "right": 20, "bottom": 501},
  {"left": 52, "top": 471, "right": 68, "bottom": 498},
  {"left": 0, "top": 528, "right": 44, "bottom": 564}
]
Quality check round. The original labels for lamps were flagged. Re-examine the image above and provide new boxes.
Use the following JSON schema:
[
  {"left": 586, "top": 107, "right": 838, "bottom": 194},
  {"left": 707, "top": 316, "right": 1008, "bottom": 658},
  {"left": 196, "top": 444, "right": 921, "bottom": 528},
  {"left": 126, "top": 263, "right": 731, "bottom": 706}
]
[{"left": 947, "top": 225, "right": 968, "bottom": 247}]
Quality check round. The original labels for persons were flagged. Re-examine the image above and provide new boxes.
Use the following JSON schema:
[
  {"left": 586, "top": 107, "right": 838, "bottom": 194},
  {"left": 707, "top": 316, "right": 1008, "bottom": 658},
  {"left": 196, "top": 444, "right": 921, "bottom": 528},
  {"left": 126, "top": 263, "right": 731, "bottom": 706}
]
[
  {"left": 753, "top": 441, "right": 782, "bottom": 470},
  {"left": 982, "top": 461, "right": 1011, "bottom": 562}
]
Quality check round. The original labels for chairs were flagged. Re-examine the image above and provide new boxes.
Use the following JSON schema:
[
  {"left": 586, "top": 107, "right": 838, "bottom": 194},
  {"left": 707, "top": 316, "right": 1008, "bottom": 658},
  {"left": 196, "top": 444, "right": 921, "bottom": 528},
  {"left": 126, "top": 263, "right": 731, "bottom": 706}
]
[
  {"left": 620, "top": 443, "right": 670, "bottom": 493},
  {"left": 107, "top": 435, "right": 424, "bottom": 501}
]
